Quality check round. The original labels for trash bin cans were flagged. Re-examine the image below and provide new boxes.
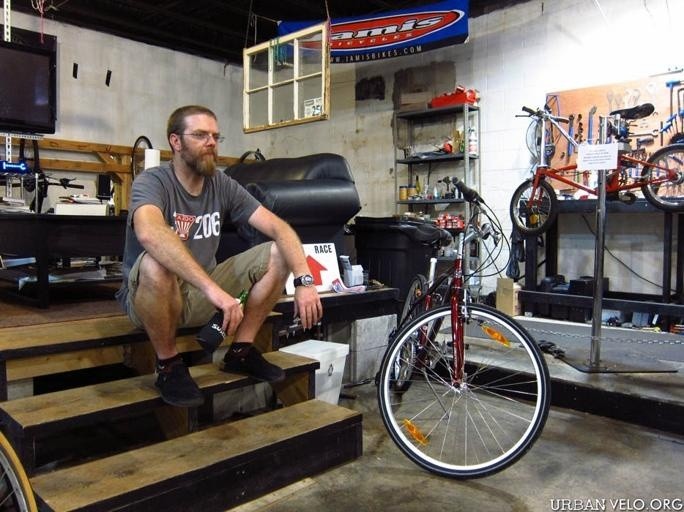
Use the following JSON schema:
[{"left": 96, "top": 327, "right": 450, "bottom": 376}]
[{"left": 279, "top": 339, "right": 350, "bottom": 407}]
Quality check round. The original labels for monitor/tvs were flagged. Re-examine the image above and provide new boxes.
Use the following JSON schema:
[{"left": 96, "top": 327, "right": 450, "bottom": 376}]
[{"left": 0, "top": 39, "right": 56, "bottom": 134}]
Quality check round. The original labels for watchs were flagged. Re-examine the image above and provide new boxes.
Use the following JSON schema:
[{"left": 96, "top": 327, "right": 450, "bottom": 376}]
[{"left": 294, "top": 274, "right": 314, "bottom": 287}]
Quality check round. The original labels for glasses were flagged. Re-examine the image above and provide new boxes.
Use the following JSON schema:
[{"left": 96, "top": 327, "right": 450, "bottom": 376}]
[{"left": 176, "top": 130, "right": 226, "bottom": 143}]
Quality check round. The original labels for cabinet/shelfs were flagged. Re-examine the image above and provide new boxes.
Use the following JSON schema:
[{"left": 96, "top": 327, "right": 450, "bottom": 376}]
[
  {"left": 392, "top": 104, "right": 481, "bottom": 303},
  {"left": 517, "top": 198, "right": 684, "bottom": 333}
]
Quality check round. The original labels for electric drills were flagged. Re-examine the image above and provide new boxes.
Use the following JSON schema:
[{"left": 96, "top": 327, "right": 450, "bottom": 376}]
[
  {"left": 636, "top": 137, "right": 654, "bottom": 151},
  {"left": 437, "top": 176, "right": 477, "bottom": 204}
]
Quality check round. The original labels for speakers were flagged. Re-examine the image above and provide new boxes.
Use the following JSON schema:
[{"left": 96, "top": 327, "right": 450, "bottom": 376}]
[{"left": 96, "top": 174, "right": 110, "bottom": 200}]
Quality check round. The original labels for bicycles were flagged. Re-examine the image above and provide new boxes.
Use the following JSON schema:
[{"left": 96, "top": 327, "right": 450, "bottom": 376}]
[
  {"left": 504, "top": 99, "right": 684, "bottom": 237},
  {"left": 0, "top": 423, "right": 41, "bottom": 512},
  {"left": 367, "top": 172, "right": 553, "bottom": 486}
]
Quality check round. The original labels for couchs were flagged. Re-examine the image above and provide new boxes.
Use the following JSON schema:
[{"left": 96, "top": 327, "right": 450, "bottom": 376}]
[{"left": 218, "top": 152, "right": 363, "bottom": 265}]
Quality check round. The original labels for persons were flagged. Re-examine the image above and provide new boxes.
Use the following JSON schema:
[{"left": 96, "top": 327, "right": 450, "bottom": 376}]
[{"left": 115, "top": 105, "right": 323, "bottom": 407}]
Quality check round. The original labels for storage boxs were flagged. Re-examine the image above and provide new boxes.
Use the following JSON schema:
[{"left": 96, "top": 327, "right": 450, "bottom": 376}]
[{"left": 210, "top": 337, "right": 350, "bottom": 419}]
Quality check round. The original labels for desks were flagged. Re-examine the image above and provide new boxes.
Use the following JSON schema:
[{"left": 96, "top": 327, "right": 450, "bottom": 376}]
[{"left": 0, "top": 213, "right": 126, "bottom": 310}]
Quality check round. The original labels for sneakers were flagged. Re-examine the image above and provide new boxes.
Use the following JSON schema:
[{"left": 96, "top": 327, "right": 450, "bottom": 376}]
[
  {"left": 218, "top": 345, "right": 286, "bottom": 383},
  {"left": 152, "top": 364, "right": 204, "bottom": 408}
]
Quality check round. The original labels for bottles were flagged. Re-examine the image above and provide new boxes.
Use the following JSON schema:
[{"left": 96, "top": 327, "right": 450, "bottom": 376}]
[
  {"left": 196, "top": 288, "right": 251, "bottom": 353},
  {"left": 399, "top": 176, "right": 421, "bottom": 200}
]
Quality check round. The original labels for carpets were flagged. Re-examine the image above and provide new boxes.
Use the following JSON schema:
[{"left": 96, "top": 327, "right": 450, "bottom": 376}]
[{"left": 439, "top": 317, "right": 684, "bottom": 364}]
